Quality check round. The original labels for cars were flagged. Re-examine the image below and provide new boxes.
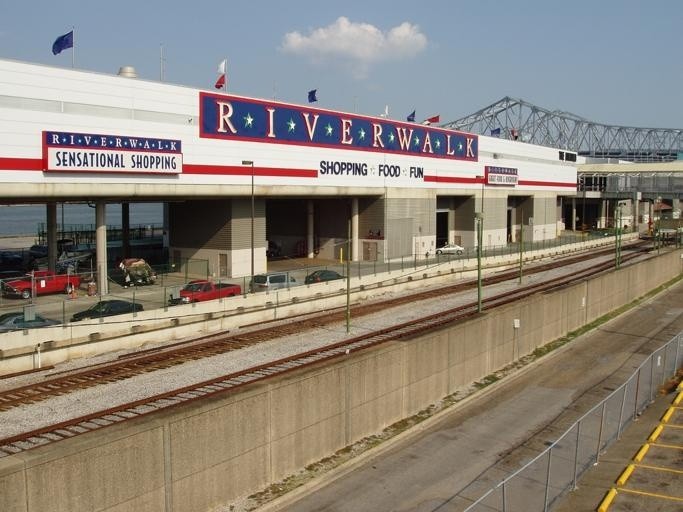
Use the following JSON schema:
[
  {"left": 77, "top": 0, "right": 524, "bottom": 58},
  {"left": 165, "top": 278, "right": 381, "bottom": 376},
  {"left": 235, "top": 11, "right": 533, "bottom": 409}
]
[
  {"left": 436, "top": 243, "right": 465, "bottom": 255},
  {"left": 69, "top": 300, "right": 144, "bottom": 322},
  {"left": 56, "top": 239, "right": 76, "bottom": 247},
  {"left": 0, "top": 312, "right": 61, "bottom": 333},
  {"left": 28, "top": 244, "right": 60, "bottom": 258},
  {"left": 304, "top": 270, "right": 347, "bottom": 285},
  {"left": 119, "top": 258, "right": 157, "bottom": 287},
  {"left": 266, "top": 248, "right": 281, "bottom": 256},
  {"left": 590, "top": 228, "right": 621, "bottom": 240}
]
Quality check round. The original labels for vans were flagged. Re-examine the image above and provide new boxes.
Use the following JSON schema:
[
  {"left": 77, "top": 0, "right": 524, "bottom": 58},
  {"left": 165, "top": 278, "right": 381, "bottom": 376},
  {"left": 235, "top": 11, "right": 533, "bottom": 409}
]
[{"left": 248, "top": 272, "right": 299, "bottom": 293}]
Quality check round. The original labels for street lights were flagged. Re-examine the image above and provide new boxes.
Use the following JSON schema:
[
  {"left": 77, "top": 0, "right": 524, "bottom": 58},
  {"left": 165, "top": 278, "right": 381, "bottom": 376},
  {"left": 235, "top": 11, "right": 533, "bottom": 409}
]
[
  {"left": 474, "top": 212, "right": 483, "bottom": 314},
  {"left": 613, "top": 205, "right": 619, "bottom": 270},
  {"left": 241, "top": 160, "right": 254, "bottom": 294},
  {"left": 475, "top": 176, "right": 484, "bottom": 259},
  {"left": 656, "top": 196, "right": 662, "bottom": 256},
  {"left": 617, "top": 203, "right": 623, "bottom": 265}
]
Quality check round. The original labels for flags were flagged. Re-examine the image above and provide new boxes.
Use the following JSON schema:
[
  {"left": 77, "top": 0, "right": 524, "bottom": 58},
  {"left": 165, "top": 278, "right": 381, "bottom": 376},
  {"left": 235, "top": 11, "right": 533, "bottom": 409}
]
[
  {"left": 307, "top": 89, "right": 318, "bottom": 104},
  {"left": 214, "top": 59, "right": 227, "bottom": 89},
  {"left": 51, "top": 30, "right": 72, "bottom": 55},
  {"left": 378, "top": 105, "right": 518, "bottom": 142}
]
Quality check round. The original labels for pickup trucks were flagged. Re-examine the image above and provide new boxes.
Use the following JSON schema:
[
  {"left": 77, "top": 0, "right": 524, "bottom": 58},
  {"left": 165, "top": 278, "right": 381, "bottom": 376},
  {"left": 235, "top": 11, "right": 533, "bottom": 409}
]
[
  {"left": 167, "top": 279, "right": 240, "bottom": 306},
  {"left": 2, "top": 270, "right": 81, "bottom": 299}
]
